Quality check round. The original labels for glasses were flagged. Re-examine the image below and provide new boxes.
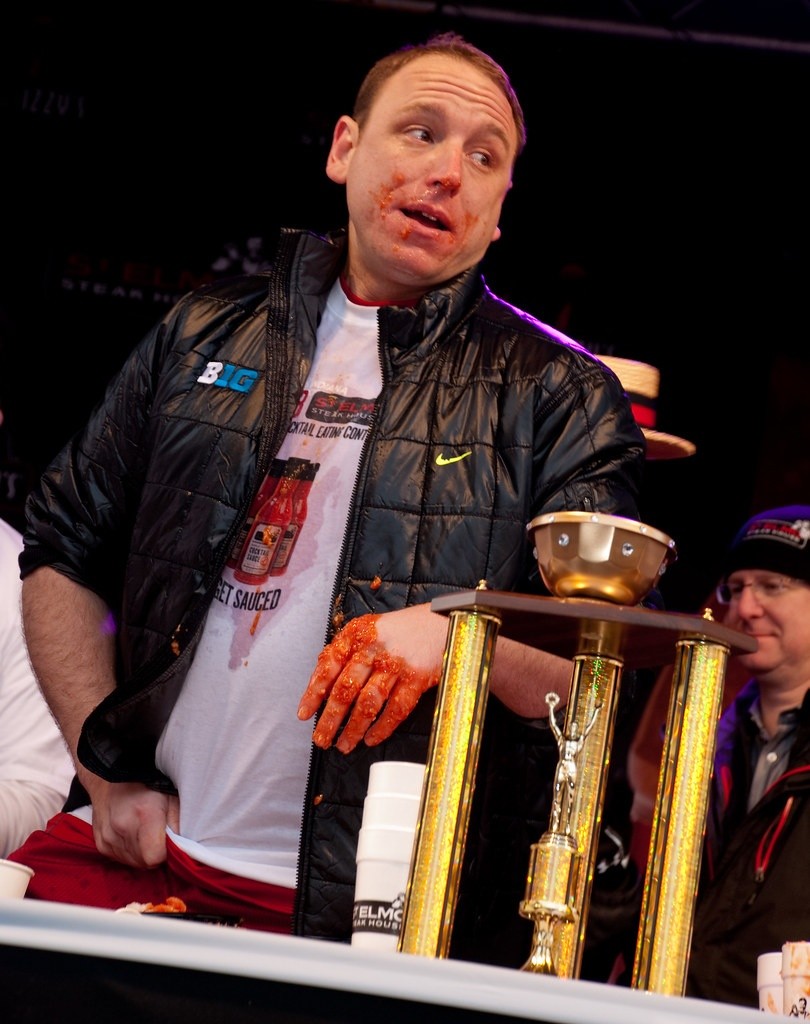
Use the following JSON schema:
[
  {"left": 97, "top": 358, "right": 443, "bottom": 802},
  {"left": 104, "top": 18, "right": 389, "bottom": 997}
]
[{"left": 716, "top": 580, "right": 806, "bottom": 602}]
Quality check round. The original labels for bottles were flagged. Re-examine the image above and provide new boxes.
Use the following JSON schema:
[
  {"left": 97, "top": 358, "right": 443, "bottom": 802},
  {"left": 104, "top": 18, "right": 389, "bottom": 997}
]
[
  {"left": 233, "top": 455, "right": 311, "bottom": 584},
  {"left": 267, "top": 462, "right": 322, "bottom": 577},
  {"left": 224, "top": 457, "right": 284, "bottom": 568}
]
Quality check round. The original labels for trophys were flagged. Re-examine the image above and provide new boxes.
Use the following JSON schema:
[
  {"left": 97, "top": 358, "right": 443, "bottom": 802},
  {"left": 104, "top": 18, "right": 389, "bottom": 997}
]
[{"left": 397, "top": 509, "right": 759, "bottom": 1000}]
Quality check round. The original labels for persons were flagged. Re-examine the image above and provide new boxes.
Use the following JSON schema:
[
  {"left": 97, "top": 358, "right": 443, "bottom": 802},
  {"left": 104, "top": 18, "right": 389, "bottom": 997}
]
[
  {"left": 451, "top": 351, "right": 696, "bottom": 983},
  {"left": 615, "top": 505, "right": 810, "bottom": 1009},
  {"left": 6, "top": 37, "right": 646, "bottom": 949},
  {"left": 1, "top": 520, "right": 75, "bottom": 864}
]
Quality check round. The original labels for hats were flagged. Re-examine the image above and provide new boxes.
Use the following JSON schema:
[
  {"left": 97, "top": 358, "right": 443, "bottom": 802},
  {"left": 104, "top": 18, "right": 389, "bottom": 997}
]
[{"left": 726, "top": 507, "right": 810, "bottom": 589}]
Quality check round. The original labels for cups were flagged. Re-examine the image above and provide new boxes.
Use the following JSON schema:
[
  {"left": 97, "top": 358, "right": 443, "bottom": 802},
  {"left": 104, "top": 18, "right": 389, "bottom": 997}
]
[
  {"left": 363, "top": 793, "right": 422, "bottom": 832},
  {"left": 756, "top": 941, "right": 810, "bottom": 1021},
  {"left": 0, "top": 855, "right": 36, "bottom": 900},
  {"left": 351, "top": 825, "right": 417, "bottom": 954},
  {"left": 366, "top": 762, "right": 427, "bottom": 797}
]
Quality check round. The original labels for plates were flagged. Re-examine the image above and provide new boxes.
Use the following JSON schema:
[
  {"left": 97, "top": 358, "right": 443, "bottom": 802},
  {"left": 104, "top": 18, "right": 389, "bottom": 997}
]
[{"left": 139, "top": 910, "right": 245, "bottom": 929}]
